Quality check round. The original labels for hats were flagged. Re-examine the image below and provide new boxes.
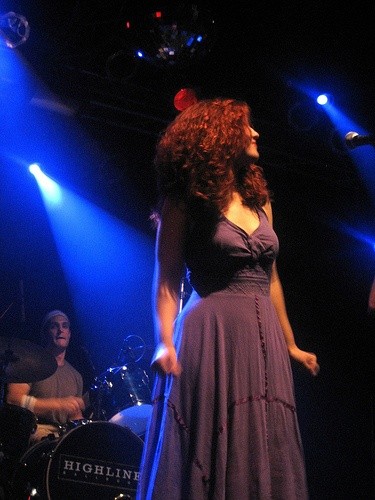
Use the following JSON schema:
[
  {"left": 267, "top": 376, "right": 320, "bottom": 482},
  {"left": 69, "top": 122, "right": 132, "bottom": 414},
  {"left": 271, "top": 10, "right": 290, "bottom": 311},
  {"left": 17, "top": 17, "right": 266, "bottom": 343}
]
[{"left": 40, "top": 311, "right": 69, "bottom": 341}]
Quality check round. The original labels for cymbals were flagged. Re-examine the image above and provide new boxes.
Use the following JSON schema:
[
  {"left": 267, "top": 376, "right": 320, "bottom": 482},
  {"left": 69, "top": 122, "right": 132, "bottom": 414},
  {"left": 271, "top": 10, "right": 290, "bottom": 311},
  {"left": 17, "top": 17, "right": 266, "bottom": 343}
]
[
  {"left": 0, "top": 336, "right": 58, "bottom": 383},
  {"left": 128, "top": 345, "right": 157, "bottom": 361}
]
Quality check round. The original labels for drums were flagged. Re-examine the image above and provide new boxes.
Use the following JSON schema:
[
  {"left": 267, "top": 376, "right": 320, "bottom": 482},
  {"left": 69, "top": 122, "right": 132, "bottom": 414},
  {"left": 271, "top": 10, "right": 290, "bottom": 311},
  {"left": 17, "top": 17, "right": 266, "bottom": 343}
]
[
  {"left": 17, "top": 420, "right": 150, "bottom": 500},
  {"left": 0, "top": 404, "right": 38, "bottom": 470},
  {"left": 89, "top": 364, "right": 155, "bottom": 437}
]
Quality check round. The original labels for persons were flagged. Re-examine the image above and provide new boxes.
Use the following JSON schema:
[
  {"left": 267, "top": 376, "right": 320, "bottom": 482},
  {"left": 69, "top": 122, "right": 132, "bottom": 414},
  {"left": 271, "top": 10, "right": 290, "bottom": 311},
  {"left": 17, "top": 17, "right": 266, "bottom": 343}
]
[
  {"left": 6, "top": 310, "right": 86, "bottom": 445},
  {"left": 149, "top": 97, "right": 319, "bottom": 500}
]
